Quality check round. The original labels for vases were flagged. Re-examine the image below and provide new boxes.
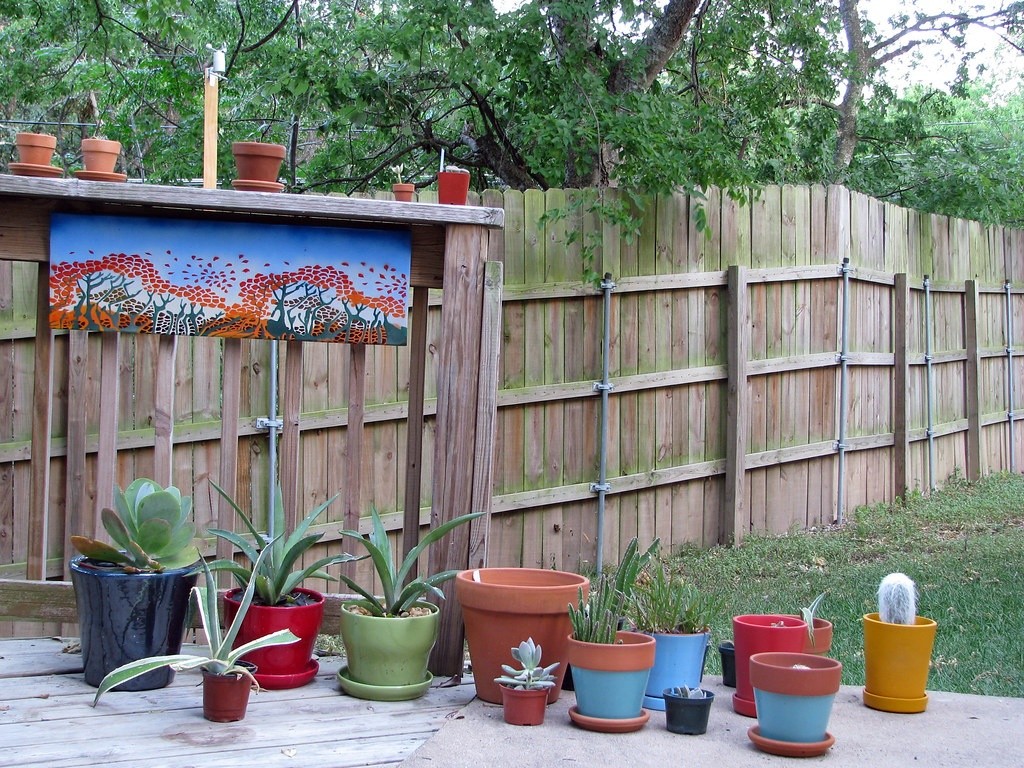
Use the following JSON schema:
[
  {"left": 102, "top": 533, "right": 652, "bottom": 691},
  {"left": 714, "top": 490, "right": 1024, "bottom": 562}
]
[
  {"left": 16, "top": 134, "right": 57, "bottom": 166},
  {"left": 456, "top": 568, "right": 590, "bottom": 705},
  {"left": 231, "top": 142, "right": 286, "bottom": 182}
]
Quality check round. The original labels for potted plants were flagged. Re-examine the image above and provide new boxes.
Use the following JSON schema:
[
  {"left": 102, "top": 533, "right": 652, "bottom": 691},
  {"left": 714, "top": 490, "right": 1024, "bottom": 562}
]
[
  {"left": 609, "top": 546, "right": 727, "bottom": 699},
  {"left": 749, "top": 652, "right": 842, "bottom": 743},
  {"left": 786, "top": 590, "right": 834, "bottom": 657},
  {"left": 494, "top": 637, "right": 561, "bottom": 725},
  {"left": 863, "top": 572, "right": 937, "bottom": 698},
  {"left": 567, "top": 573, "right": 656, "bottom": 719},
  {"left": 389, "top": 163, "right": 415, "bottom": 202},
  {"left": 339, "top": 505, "right": 487, "bottom": 687},
  {"left": 91, "top": 547, "right": 302, "bottom": 723},
  {"left": 68, "top": 478, "right": 207, "bottom": 691},
  {"left": 562, "top": 537, "right": 661, "bottom": 691},
  {"left": 82, "top": 136, "right": 121, "bottom": 172},
  {"left": 435, "top": 166, "right": 470, "bottom": 205},
  {"left": 663, "top": 685, "right": 715, "bottom": 733},
  {"left": 183, "top": 478, "right": 373, "bottom": 674},
  {"left": 718, "top": 640, "right": 736, "bottom": 688},
  {"left": 732, "top": 615, "right": 808, "bottom": 702}
]
